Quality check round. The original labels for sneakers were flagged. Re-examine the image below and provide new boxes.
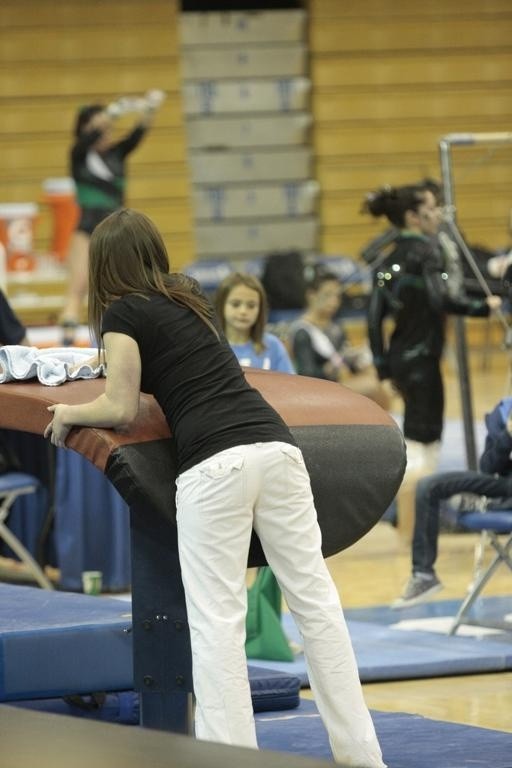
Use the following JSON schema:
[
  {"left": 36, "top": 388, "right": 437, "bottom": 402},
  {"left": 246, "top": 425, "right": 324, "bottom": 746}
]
[{"left": 391, "top": 571, "right": 445, "bottom": 612}]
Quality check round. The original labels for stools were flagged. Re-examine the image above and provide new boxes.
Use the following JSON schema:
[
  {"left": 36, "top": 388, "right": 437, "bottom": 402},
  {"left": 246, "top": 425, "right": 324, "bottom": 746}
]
[{"left": 448, "top": 511, "right": 511, "bottom": 636}]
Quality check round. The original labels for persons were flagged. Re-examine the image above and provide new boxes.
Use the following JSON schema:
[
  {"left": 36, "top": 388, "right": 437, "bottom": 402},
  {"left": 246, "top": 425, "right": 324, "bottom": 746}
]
[
  {"left": 0, "top": 289, "right": 61, "bottom": 582},
  {"left": 41, "top": 209, "right": 385, "bottom": 768}
]
